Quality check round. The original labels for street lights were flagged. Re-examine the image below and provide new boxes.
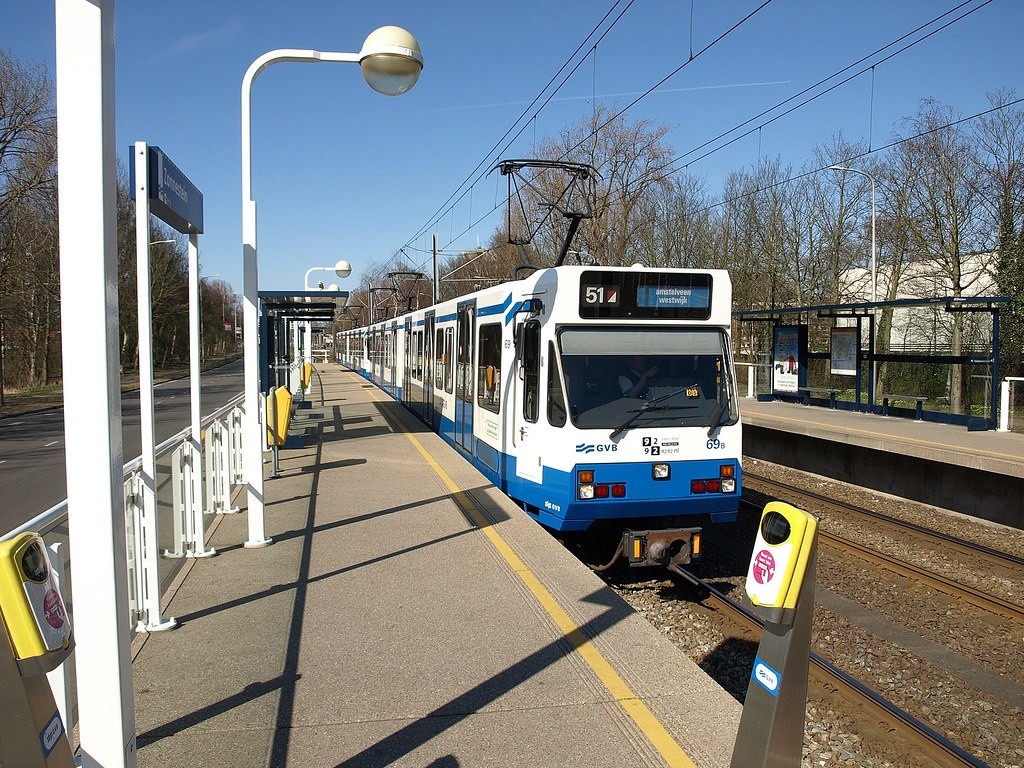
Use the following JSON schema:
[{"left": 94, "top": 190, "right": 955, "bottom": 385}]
[
  {"left": 242, "top": 26, "right": 422, "bottom": 549},
  {"left": 302, "top": 260, "right": 352, "bottom": 395},
  {"left": 829, "top": 165, "right": 877, "bottom": 406},
  {"left": 199, "top": 274, "right": 220, "bottom": 367}
]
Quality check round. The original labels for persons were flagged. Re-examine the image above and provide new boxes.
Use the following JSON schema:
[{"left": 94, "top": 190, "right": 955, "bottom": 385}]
[
  {"left": 619, "top": 355, "right": 670, "bottom": 398},
  {"left": 487, "top": 344, "right": 499, "bottom": 369}
]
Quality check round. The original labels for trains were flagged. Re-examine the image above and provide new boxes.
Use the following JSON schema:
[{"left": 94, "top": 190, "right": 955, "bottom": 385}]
[{"left": 332, "top": 158, "right": 744, "bottom": 587}]
